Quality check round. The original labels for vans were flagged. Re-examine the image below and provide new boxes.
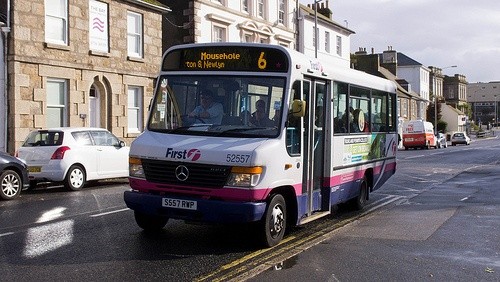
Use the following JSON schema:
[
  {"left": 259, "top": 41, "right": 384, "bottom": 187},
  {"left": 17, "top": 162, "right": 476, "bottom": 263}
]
[{"left": 403, "top": 120, "right": 438, "bottom": 150}]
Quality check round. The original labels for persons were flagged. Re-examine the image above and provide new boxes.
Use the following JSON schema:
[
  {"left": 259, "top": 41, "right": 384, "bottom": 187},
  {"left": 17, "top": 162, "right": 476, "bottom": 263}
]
[
  {"left": 177, "top": 90, "right": 224, "bottom": 126},
  {"left": 250, "top": 99, "right": 281, "bottom": 128},
  {"left": 334, "top": 107, "right": 390, "bottom": 133}
]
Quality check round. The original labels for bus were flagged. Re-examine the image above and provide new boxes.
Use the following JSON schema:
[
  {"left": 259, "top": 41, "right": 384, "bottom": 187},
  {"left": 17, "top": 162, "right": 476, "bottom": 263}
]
[{"left": 124, "top": 42, "right": 398, "bottom": 247}]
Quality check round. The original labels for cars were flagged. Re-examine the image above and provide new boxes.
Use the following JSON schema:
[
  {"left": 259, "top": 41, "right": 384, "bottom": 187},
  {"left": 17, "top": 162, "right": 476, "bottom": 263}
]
[
  {"left": 0, "top": 151, "right": 30, "bottom": 200},
  {"left": 435, "top": 133, "right": 447, "bottom": 148},
  {"left": 451, "top": 132, "right": 471, "bottom": 145},
  {"left": 16, "top": 127, "right": 131, "bottom": 192}
]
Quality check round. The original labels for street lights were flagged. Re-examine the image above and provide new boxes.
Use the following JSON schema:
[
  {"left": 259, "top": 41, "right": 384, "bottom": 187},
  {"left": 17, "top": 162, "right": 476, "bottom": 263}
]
[{"left": 434, "top": 66, "right": 457, "bottom": 135}]
